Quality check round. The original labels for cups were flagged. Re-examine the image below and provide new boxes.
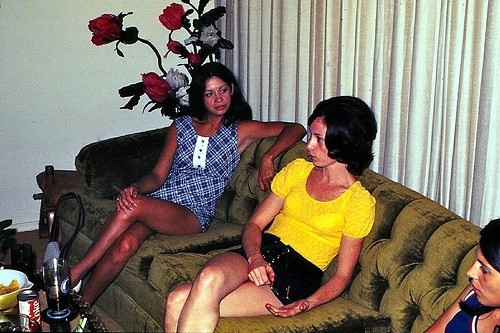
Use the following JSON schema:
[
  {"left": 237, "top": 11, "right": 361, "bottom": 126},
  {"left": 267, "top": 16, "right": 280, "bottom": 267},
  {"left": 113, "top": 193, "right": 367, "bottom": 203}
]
[
  {"left": 17, "top": 289, "right": 41, "bottom": 333},
  {"left": 43, "top": 258, "right": 70, "bottom": 319}
]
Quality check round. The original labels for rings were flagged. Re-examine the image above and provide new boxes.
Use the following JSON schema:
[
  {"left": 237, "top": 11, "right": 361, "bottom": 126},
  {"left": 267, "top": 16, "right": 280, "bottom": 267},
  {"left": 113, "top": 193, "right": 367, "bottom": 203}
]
[{"left": 116, "top": 198, "right": 119, "bottom": 201}]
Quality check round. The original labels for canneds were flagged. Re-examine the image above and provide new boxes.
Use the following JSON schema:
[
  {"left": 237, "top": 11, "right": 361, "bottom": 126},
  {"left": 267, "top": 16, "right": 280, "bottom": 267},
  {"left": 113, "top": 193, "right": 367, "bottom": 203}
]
[{"left": 17, "top": 289, "right": 42, "bottom": 333}]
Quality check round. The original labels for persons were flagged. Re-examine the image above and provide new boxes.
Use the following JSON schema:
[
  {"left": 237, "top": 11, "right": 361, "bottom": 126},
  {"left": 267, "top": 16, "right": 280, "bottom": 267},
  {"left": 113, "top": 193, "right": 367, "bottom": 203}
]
[
  {"left": 70, "top": 63, "right": 307, "bottom": 306},
  {"left": 167, "top": 96, "right": 377, "bottom": 333},
  {"left": 422, "top": 219, "right": 500, "bottom": 333}
]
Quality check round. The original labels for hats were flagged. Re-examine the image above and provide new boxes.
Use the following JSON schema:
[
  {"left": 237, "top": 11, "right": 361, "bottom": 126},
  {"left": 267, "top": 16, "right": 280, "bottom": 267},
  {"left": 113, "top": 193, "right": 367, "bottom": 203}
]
[{"left": 480, "top": 218, "right": 500, "bottom": 273}]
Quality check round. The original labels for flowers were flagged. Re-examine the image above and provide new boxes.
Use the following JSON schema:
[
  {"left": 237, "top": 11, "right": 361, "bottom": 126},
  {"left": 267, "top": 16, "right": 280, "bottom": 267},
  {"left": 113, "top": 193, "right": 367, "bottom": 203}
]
[{"left": 88, "top": 0, "right": 234, "bottom": 120}]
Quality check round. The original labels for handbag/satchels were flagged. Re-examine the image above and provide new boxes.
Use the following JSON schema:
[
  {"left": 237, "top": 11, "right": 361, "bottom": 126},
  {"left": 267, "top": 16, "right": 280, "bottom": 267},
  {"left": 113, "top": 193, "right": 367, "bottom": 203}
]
[{"left": 43, "top": 242, "right": 59, "bottom": 272}]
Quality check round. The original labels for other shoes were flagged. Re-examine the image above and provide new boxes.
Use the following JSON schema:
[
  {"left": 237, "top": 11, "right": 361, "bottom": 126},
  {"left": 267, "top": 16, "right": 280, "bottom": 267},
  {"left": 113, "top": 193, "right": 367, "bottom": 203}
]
[{"left": 73, "top": 279, "right": 82, "bottom": 293}]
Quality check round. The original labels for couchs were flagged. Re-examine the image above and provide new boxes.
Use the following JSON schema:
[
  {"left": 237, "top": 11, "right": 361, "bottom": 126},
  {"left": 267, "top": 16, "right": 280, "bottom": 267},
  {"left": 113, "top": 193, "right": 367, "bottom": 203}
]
[{"left": 49, "top": 126, "right": 481, "bottom": 333}]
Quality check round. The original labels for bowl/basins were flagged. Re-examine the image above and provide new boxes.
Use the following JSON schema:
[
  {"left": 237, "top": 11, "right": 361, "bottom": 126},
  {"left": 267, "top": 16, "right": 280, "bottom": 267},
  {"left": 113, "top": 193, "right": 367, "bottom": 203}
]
[{"left": 0, "top": 269, "right": 34, "bottom": 312}]
